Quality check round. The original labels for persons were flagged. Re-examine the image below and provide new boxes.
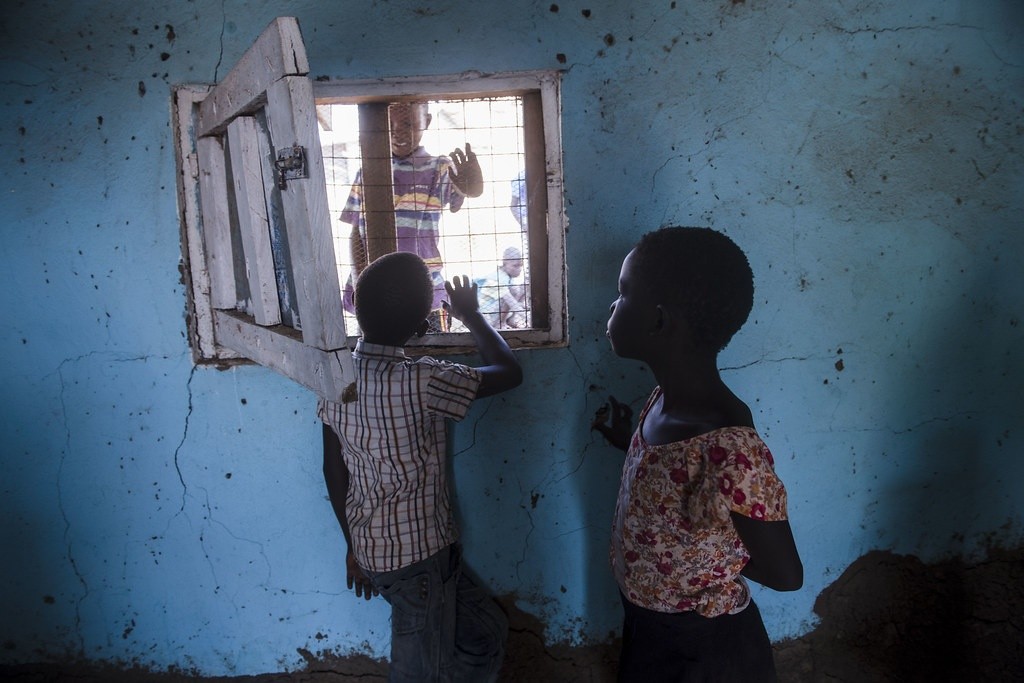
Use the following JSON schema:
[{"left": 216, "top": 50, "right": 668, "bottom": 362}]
[
  {"left": 339, "top": 101, "right": 484, "bottom": 333},
  {"left": 479, "top": 247, "right": 530, "bottom": 328},
  {"left": 317, "top": 250, "right": 524, "bottom": 683},
  {"left": 511, "top": 170, "right": 530, "bottom": 307},
  {"left": 593, "top": 225, "right": 804, "bottom": 683}
]
